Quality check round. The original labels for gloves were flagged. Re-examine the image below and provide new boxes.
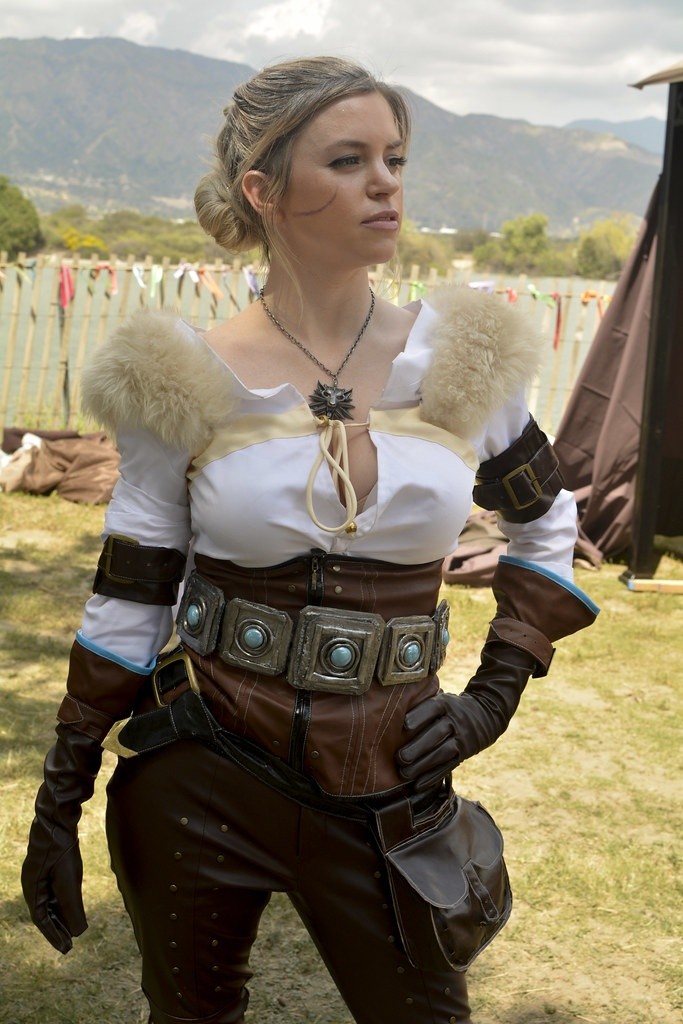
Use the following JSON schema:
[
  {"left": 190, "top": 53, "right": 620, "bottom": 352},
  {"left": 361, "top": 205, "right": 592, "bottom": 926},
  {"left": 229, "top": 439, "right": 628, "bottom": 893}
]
[
  {"left": 399, "top": 554, "right": 600, "bottom": 791},
  {"left": 21, "top": 630, "right": 157, "bottom": 957}
]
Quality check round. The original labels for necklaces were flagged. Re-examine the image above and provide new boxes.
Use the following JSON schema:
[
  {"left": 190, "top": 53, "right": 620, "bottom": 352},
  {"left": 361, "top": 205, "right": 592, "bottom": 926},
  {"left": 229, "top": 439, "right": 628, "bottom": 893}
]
[{"left": 259, "top": 282, "right": 376, "bottom": 422}]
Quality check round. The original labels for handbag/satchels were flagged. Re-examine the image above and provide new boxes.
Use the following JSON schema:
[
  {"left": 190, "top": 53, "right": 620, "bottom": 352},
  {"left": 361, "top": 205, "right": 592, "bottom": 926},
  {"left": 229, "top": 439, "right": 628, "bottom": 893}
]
[{"left": 378, "top": 797, "right": 513, "bottom": 973}]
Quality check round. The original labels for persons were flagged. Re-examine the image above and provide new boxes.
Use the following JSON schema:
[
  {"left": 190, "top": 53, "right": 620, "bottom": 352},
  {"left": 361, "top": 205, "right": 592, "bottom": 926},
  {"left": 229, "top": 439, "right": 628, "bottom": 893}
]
[{"left": 19, "top": 55, "right": 602, "bottom": 1024}]
